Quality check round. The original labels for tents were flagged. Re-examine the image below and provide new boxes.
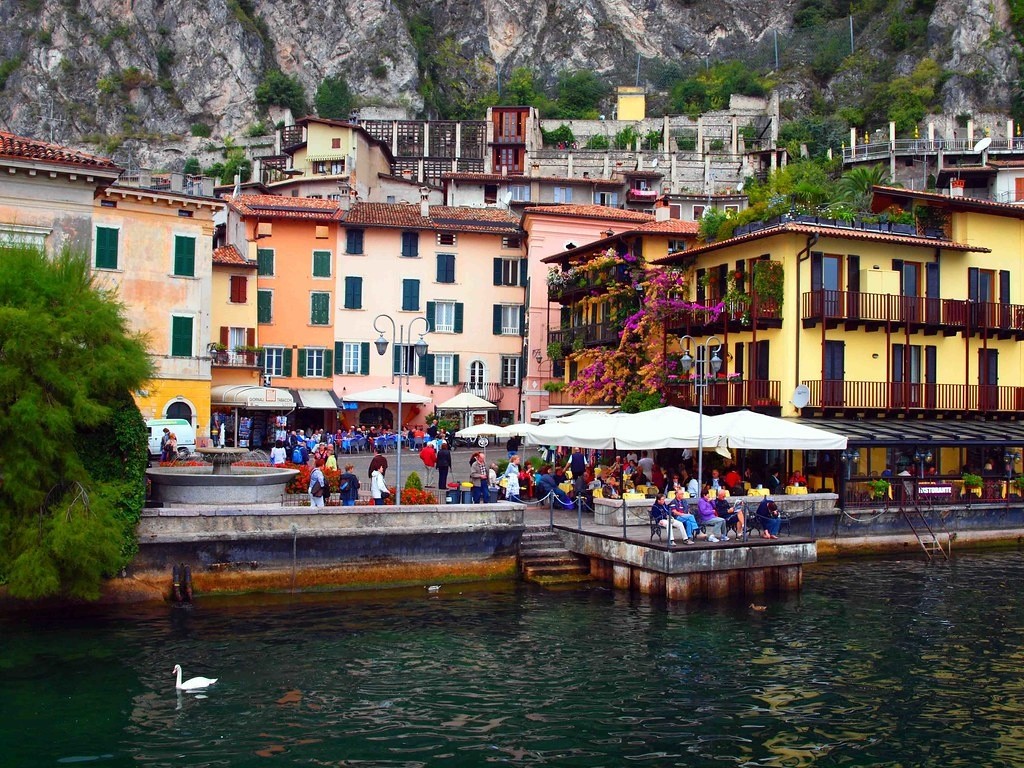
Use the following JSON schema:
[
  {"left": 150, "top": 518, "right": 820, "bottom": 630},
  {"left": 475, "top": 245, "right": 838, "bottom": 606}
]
[{"left": 526, "top": 407, "right": 847, "bottom": 546}]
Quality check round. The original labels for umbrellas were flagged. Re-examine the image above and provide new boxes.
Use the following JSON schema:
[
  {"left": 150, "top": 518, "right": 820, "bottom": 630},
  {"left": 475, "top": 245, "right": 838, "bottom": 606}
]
[
  {"left": 496, "top": 423, "right": 538, "bottom": 466},
  {"left": 455, "top": 423, "right": 502, "bottom": 461},
  {"left": 437, "top": 392, "right": 497, "bottom": 425}
]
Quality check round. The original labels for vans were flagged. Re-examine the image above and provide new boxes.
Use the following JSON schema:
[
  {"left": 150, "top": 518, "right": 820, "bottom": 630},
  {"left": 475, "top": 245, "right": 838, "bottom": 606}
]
[{"left": 145, "top": 419, "right": 198, "bottom": 461}]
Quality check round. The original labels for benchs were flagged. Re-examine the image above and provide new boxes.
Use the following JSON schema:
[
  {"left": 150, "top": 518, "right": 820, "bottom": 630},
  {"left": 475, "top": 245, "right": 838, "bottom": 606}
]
[{"left": 648, "top": 508, "right": 791, "bottom": 542}]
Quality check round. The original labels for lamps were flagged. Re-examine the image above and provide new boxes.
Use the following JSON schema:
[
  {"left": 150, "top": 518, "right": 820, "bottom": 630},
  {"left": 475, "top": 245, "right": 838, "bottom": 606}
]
[{"left": 207, "top": 342, "right": 217, "bottom": 359}]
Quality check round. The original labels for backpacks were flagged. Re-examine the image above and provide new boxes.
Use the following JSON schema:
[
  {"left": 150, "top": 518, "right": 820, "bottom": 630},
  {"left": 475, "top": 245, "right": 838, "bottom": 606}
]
[
  {"left": 292, "top": 445, "right": 304, "bottom": 463},
  {"left": 340, "top": 475, "right": 352, "bottom": 492}
]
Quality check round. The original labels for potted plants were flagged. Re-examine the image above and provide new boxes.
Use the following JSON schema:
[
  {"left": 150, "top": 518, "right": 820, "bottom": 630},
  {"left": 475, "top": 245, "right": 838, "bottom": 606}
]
[
  {"left": 962, "top": 473, "right": 984, "bottom": 489},
  {"left": 697, "top": 208, "right": 726, "bottom": 242},
  {"left": 868, "top": 479, "right": 890, "bottom": 499},
  {"left": 235, "top": 345, "right": 263, "bottom": 355},
  {"left": 795, "top": 201, "right": 916, "bottom": 235},
  {"left": 216, "top": 344, "right": 228, "bottom": 361},
  {"left": 720, "top": 203, "right": 790, "bottom": 236}
]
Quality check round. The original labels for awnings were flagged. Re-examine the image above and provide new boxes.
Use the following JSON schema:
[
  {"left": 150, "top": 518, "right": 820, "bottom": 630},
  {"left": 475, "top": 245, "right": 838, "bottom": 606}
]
[
  {"left": 533, "top": 409, "right": 617, "bottom": 420},
  {"left": 344, "top": 388, "right": 433, "bottom": 405},
  {"left": 291, "top": 389, "right": 343, "bottom": 411}
]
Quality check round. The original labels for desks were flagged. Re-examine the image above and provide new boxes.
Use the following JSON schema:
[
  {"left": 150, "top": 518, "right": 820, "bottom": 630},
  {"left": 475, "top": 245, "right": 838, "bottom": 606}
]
[
  {"left": 339, "top": 438, "right": 355, "bottom": 450},
  {"left": 636, "top": 484, "right": 659, "bottom": 499},
  {"left": 740, "top": 481, "right": 751, "bottom": 492},
  {"left": 559, "top": 483, "right": 576, "bottom": 496},
  {"left": 747, "top": 488, "right": 770, "bottom": 496},
  {"left": 708, "top": 489, "right": 731, "bottom": 498},
  {"left": 784, "top": 486, "right": 808, "bottom": 495},
  {"left": 594, "top": 488, "right": 645, "bottom": 500},
  {"left": 373, "top": 436, "right": 388, "bottom": 440},
  {"left": 304, "top": 438, "right": 316, "bottom": 453},
  {"left": 668, "top": 491, "right": 690, "bottom": 499}
]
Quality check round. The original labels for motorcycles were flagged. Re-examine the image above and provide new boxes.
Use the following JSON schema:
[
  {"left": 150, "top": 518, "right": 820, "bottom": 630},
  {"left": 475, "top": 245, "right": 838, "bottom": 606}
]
[{"left": 450, "top": 430, "right": 490, "bottom": 448}]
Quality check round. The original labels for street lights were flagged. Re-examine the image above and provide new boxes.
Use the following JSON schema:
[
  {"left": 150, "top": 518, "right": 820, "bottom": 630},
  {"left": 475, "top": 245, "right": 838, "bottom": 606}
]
[
  {"left": 678, "top": 334, "right": 724, "bottom": 497},
  {"left": 372, "top": 313, "right": 430, "bottom": 505}
]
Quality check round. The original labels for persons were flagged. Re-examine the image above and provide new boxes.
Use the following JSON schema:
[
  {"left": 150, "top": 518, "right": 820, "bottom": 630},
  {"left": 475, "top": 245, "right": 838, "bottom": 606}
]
[
  {"left": 270, "top": 420, "right": 453, "bottom": 507},
  {"left": 469, "top": 435, "right": 806, "bottom": 547},
  {"left": 161, "top": 426, "right": 179, "bottom": 463},
  {"left": 1005, "top": 465, "right": 1017, "bottom": 500},
  {"left": 983, "top": 464, "right": 994, "bottom": 503},
  {"left": 882, "top": 466, "right": 942, "bottom": 508}
]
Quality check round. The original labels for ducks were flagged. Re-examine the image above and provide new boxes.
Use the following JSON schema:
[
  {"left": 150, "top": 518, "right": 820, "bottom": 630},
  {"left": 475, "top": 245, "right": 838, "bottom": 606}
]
[{"left": 172, "top": 664, "right": 218, "bottom": 690}]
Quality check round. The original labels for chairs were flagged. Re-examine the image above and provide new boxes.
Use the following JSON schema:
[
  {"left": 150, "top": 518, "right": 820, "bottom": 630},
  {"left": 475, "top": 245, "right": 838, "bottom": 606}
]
[
  {"left": 293, "top": 433, "right": 447, "bottom": 455},
  {"left": 499, "top": 467, "right": 813, "bottom": 509}
]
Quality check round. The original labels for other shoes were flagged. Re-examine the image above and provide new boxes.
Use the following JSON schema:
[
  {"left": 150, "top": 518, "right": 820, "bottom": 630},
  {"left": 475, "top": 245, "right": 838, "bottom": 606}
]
[
  {"left": 721, "top": 536, "right": 731, "bottom": 542},
  {"left": 688, "top": 540, "right": 695, "bottom": 544},
  {"left": 742, "top": 527, "right": 750, "bottom": 533},
  {"left": 735, "top": 535, "right": 748, "bottom": 541},
  {"left": 697, "top": 533, "right": 708, "bottom": 539},
  {"left": 684, "top": 540, "right": 692, "bottom": 545},
  {"left": 670, "top": 541, "right": 677, "bottom": 547},
  {"left": 708, "top": 536, "right": 720, "bottom": 542}
]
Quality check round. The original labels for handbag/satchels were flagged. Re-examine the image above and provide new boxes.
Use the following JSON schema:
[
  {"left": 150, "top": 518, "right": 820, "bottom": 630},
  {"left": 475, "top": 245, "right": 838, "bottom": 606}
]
[{"left": 382, "top": 492, "right": 390, "bottom": 499}]
